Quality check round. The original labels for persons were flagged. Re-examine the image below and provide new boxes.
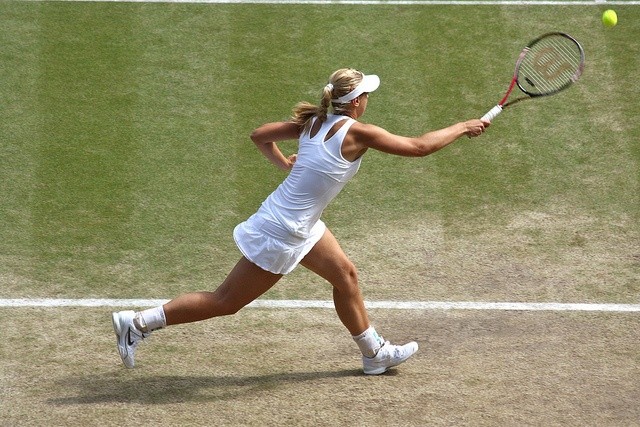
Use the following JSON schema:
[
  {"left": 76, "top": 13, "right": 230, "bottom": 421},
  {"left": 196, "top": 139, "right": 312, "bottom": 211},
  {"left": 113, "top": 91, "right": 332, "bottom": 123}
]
[{"left": 113, "top": 67, "right": 492, "bottom": 376}]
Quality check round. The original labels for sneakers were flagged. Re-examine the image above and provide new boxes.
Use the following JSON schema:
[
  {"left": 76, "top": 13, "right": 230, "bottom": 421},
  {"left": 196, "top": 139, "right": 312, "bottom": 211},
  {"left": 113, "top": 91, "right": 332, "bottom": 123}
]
[
  {"left": 362, "top": 335, "right": 419, "bottom": 374},
  {"left": 112, "top": 309, "right": 149, "bottom": 369}
]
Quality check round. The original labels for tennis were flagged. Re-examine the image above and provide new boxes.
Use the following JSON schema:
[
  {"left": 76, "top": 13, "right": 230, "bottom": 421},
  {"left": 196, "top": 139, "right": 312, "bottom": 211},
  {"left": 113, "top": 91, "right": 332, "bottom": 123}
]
[{"left": 602, "top": 9, "right": 618, "bottom": 29}]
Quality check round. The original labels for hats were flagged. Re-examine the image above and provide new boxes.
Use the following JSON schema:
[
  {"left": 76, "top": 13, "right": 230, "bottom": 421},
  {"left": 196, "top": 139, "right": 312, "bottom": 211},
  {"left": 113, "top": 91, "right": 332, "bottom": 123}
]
[{"left": 331, "top": 71, "right": 380, "bottom": 104}]
